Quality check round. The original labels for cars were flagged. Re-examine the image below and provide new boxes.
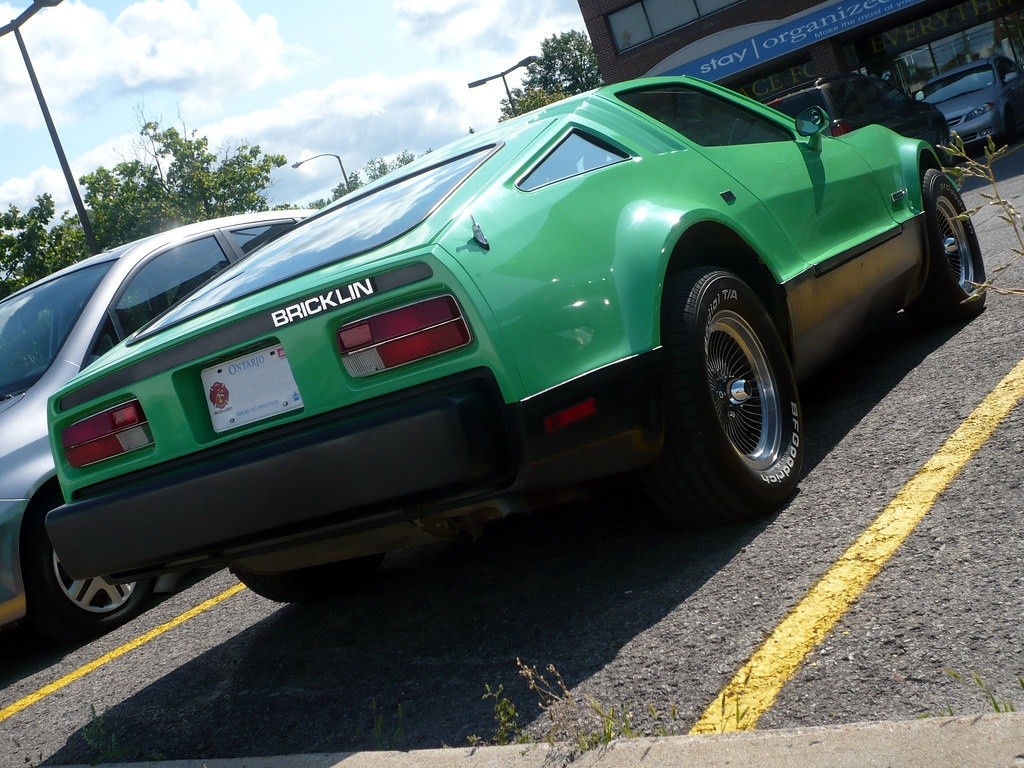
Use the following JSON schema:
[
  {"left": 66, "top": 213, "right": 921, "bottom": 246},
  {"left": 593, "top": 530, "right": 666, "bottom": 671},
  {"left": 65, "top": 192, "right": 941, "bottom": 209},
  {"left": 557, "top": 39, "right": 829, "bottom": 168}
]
[
  {"left": 920, "top": 56, "right": 1024, "bottom": 146},
  {"left": 756, "top": 73, "right": 951, "bottom": 173},
  {"left": 0, "top": 208, "right": 319, "bottom": 625}
]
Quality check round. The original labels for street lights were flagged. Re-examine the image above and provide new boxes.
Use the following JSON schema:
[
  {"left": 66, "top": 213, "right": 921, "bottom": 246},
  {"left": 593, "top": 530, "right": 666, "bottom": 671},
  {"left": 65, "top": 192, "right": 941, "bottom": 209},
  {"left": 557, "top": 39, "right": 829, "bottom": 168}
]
[
  {"left": 468, "top": 55, "right": 538, "bottom": 118},
  {"left": 291, "top": 154, "right": 350, "bottom": 191},
  {"left": 0, "top": 0, "right": 99, "bottom": 254}
]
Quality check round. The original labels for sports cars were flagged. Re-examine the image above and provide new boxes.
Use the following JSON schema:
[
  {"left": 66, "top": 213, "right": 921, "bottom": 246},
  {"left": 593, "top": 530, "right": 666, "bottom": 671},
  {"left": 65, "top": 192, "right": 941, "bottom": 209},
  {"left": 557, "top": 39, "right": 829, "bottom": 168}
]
[{"left": 47, "top": 73, "right": 988, "bottom": 606}]
